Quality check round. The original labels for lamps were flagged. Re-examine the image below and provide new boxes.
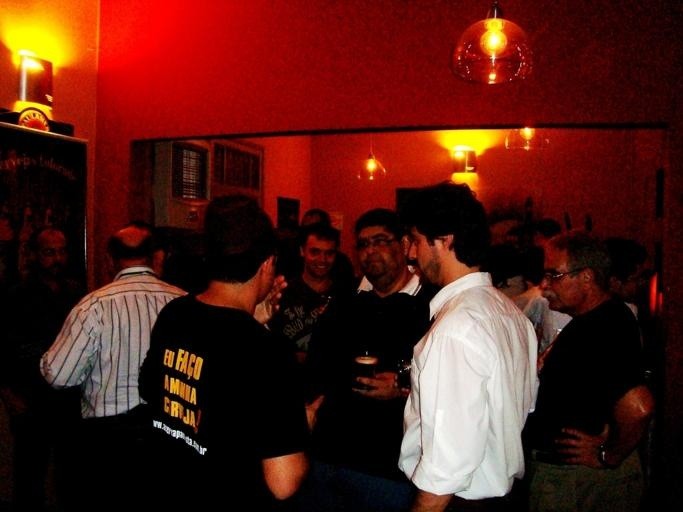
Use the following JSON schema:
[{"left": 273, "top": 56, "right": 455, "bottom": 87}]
[
  {"left": 12, "top": 53, "right": 53, "bottom": 132},
  {"left": 505, "top": 126, "right": 552, "bottom": 153},
  {"left": 350, "top": 141, "right": 387, "bottom": 184},
  {"left": 450, "top": 3, "right": 535, "bottom": 86},
  {"left": 450, "top": 146, "right": 477, "bottom": 174}
]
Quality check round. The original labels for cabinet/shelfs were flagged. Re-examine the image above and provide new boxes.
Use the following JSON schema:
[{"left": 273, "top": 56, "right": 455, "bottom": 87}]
[{"left": 1, "top": 119, "right": 89, "bottom": 287}]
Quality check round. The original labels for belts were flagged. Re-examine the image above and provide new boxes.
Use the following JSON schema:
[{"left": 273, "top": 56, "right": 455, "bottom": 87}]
[{"left": 528, "top": 450, "right": 574, "bottom": 465}]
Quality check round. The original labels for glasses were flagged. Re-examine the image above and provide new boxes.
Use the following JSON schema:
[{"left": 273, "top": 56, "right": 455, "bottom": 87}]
[{"left": 542, "top": 267, "right": 584, "bottom": 280}]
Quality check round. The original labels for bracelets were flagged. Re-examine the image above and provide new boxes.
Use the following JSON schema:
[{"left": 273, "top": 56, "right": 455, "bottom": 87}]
[{"left": 596, "top": 443, "right": 615, "bottom": 469}]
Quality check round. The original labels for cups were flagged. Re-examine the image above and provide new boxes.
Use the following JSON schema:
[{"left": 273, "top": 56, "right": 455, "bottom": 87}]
[{"left": 355, "top": 356, "right": 378, "bottom": 390}]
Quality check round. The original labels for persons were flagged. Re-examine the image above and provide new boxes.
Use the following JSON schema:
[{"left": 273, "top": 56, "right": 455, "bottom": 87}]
[
  {"left": 0, "top": 227, "right": 92, "bottom": 512},
  {"left": 399, "top": 180, "right": 539, "bottom": 512},
  {"left": 320, "top": 207, "right": 440, "bottom": 511},
  {"left": 266, "top": 224, "right": 344, "bottom": 362},
  {"left": 297, "top": 209, "right": 354, "bottom": 289},
  {"left": 522, "top": 230, "right": 657, "bottom": 511},
  {"left": 523, "top": 235, "right": 648, "bottom": 353},
  {"left": 40, "top": 224, "right": 192, "bottom": 423},
  {"left": 138, "top": 197, "right": 325, "bottom": 512}
]
